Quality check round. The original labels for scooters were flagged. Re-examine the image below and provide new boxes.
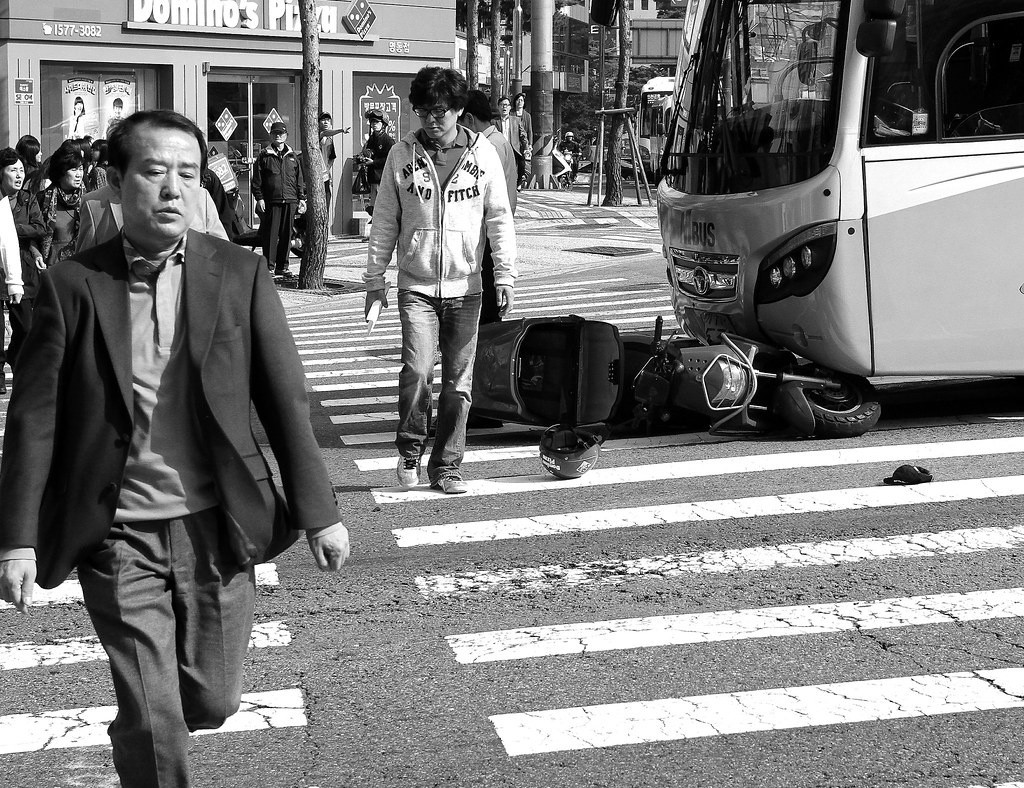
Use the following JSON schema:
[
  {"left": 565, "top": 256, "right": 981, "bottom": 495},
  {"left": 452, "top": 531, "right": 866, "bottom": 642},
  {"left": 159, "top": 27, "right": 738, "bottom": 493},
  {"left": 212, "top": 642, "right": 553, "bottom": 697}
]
[
  {"left": 224, "top": 147, "right": 308, "bottom": 259},
  {"left": 561, "top": 151, "right": 583, "bottom": 188},
  {"left": 475, "top": 314, "right": 884, "bottom": 439}
]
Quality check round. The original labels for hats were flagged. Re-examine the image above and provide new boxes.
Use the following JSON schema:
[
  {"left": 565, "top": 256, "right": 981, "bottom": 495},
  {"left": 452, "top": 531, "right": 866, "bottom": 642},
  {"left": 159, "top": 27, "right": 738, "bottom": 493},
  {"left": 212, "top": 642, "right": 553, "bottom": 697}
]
[
  {"left": 270, "top": 122, "right": 288, "bottom": 134},
  {"left": 319, "top": 111, "right": 332, "bottom": 120}
]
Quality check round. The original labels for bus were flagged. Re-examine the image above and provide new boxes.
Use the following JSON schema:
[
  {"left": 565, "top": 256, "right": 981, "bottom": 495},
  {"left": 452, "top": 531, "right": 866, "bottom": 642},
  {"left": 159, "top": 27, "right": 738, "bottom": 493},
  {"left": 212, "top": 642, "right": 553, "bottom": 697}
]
[
  {"left": 620, "top": 77, "right": 676, "bottom": 184},
  {"left": 654, "top": 1, "right": 1024, "bottom": 383}
]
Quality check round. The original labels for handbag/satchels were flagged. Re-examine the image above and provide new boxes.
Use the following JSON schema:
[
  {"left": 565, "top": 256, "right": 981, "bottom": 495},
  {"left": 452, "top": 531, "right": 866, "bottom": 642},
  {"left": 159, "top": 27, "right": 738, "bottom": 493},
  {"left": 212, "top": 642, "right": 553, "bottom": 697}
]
[{"left": 352, "top": 165, "right": 371, "bottom": 194}]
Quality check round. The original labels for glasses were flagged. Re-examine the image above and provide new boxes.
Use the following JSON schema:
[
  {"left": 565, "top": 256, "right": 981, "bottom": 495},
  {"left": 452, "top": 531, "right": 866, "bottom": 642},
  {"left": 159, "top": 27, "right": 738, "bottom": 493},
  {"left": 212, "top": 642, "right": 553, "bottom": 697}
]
[
  {"left": 499, "top": 104, "right": 512, "bottom": 108},
  {"left": 364, "top": 109, "right": 383, "bottom": 120},
  {"left": 411, "top": 107, "right": 453, "bottom": 118}
]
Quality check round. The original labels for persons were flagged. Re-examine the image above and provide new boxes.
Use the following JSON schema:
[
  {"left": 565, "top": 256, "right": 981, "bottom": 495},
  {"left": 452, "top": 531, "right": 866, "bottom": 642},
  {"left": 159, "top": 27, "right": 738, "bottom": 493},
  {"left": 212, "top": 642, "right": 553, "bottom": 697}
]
[
  {"left": 0, "top": 147, "right": 47, "bottom": 364},
  {"left": 85, "top": 138, "right": 111, "bottom": 192},
  {"left": 509, "top": 91, "right": 533, "bottom": 192},
  {"left": 319, "top": 111, "right": 350, "bottom": 212},
  {"left": 14, "top": 135, "right": 44, "bottom": 195},
  {"left": 352, "top": 109, "right": 396, "bottom": 243},
  {"left": 102, "top": 97, "right": 124, "bottom": 139},
  {"left": 1, "top": 189, "right": 24, "bottom": 395},
  {"left": 558, "top": 131, "right": 581, "bottom": 182},
  {"left": 362, "top": 66, "right": 517, "bottom": 493},
  {"left": 249, "top": 122, "right": 307, "bottom": 279},
  {"left": 490, "top": 96, "right": 532, "bottom": 167},
  {"left": 67, "top": 96, "right": 86, "bottom": 141},
  {"left": 75, "top": 177, "right": 229, "bottom": 255},
  {"left": 41, "top": 136, "right": 94, "bottom": 191},
  {"left": 29, "top": 152, "right": 88, "bottom": 273},
  {"left": 1, "top": 110, "right": 350, "bottom": 788},
  {"left": 434, "top": 88, "right": 517, "bottom": 438}
]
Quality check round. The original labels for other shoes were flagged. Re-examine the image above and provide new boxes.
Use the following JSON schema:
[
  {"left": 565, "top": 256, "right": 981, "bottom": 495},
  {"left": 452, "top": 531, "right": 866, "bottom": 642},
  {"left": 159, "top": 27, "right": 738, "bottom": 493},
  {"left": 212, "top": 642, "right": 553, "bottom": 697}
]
[{"left": 268, "top": 267, "right": 293, "bottom": 276}]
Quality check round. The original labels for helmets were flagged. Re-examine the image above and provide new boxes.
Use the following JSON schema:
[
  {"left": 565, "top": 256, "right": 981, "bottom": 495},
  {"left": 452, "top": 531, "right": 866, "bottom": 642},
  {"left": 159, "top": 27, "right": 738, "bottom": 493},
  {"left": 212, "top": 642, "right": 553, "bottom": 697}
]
[
  {"left": 368, "top": 109, "right": 389, "bottom": 126},
  {"left": 539, "top": 423, "right": 601, "bottom": 479},
  {"left": 564, "top": 132, "right": 574, "bottom": 140}
]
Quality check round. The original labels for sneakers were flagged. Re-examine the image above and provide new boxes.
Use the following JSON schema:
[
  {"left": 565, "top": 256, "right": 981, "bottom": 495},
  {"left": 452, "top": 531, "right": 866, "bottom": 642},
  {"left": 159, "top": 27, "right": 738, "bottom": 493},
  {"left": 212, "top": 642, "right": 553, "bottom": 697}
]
[
  {"left": 396, "top": 454, "right": 421, "bottom": 488},
  {"left": 436, "top": 471, "right": 468, "bottom": 493}
]
